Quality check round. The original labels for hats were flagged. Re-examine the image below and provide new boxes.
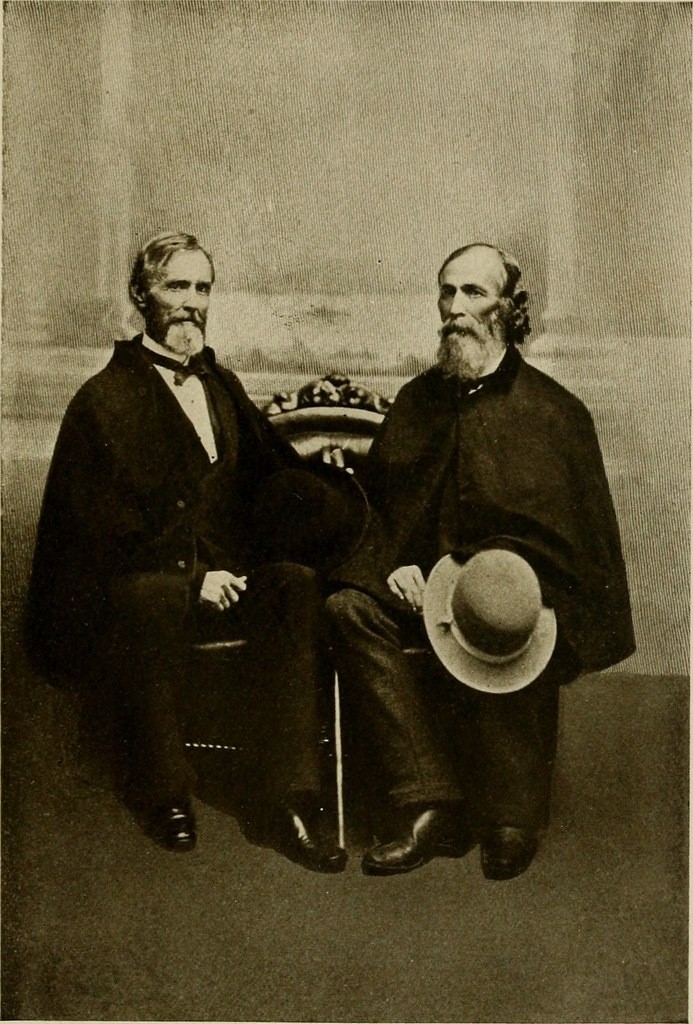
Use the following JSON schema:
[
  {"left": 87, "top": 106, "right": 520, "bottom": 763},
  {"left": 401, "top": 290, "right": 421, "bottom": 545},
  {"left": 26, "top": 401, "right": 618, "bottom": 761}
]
[{"left": 423, "top": 549, "right": 557, "bottom": 694}]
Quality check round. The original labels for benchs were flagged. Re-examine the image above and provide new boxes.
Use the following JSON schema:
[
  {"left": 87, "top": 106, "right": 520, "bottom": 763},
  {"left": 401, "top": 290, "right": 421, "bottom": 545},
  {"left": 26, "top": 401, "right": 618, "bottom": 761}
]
[{"left": 131, "top": 367, "right": 529, "bottom": 794}]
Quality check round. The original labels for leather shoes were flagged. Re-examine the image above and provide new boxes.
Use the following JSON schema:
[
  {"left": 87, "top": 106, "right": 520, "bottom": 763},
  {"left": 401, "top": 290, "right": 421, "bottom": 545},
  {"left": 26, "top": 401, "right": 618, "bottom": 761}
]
[
  {"left": 480, "top": 824, "right": 537, "bottom": 880},
  {"left": 140, "top": 790, "right": 198, "bottom": 853},
  {"left": 360, "top": 806, "right": 475, "bottom": 876},
  {"left": 265, "top": 806, "right": 348, "bottom": 873}
]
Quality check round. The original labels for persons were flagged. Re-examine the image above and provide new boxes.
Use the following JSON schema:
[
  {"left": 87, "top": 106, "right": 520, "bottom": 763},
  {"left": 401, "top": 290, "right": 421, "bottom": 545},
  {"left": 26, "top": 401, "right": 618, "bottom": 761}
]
[
  {"left": 322, "top": 242, "right": 639, "bottom": 879},
  {"left": 27, "top": 229, "right": 371, "bottom": 875}
]
